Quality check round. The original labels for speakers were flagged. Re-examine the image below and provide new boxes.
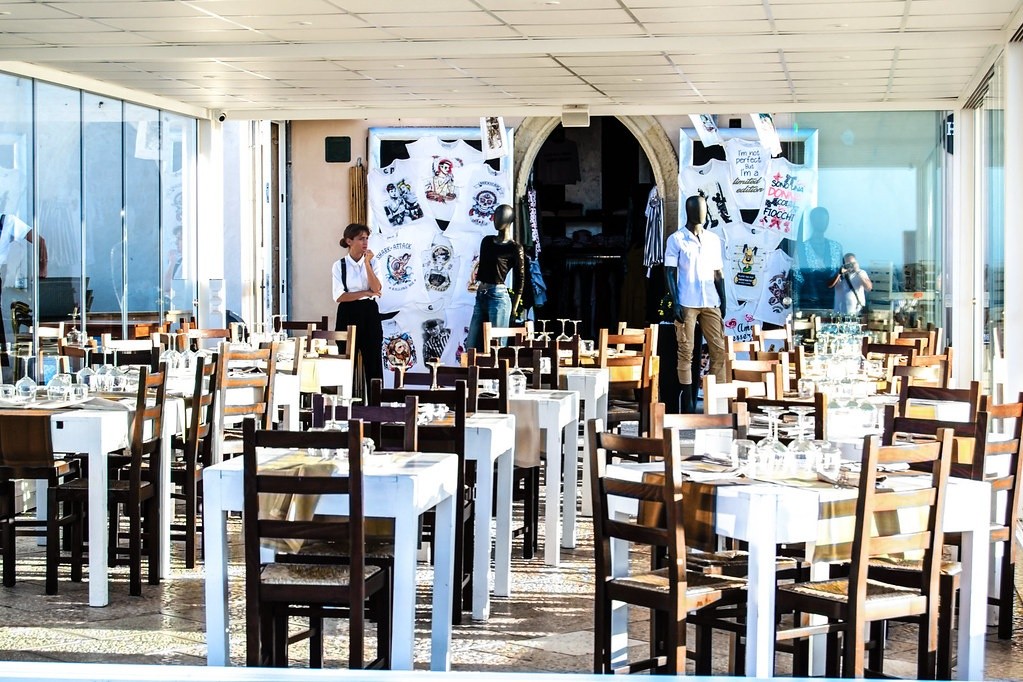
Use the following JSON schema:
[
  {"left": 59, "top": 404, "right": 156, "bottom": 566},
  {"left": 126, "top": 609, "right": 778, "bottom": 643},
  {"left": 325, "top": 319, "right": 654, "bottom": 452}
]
[{"left": 561, "top": 109, "right": 590, "bottom": 127}]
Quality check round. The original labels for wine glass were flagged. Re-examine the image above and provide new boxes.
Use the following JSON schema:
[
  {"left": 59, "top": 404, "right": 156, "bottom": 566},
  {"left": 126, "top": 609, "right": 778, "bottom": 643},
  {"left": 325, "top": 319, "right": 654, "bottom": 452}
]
[
  {"left": 748, "top": 322, "right": 868, "bottom": 480},
  {"left": 425, "top": 362, "right": 446, "bottom": 421},
  {"left": 338, "top": 397, "right": 362, "bottom": 460},
  {"left": 521, "top": 317, "right": 582, "bottom": 348},
  {"left": 1, "top": 314, "right": 287, "bottom": 407},
  {"left": 506, "top": 345, "right": 526, "bottom": 375},
  {"left": 390, "top": 365, "right": 412, "bottom": 424},
  {"left": 321, "top": 396, "right": 342, "bottom": 458},
  {"left": 487, "top": 345, "right": 507, "bottom": 369}
]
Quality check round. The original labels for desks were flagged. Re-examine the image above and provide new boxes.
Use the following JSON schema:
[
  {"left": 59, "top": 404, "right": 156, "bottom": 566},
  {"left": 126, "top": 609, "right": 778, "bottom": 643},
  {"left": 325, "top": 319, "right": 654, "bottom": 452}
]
[
  {"left": 228, "top": 354, "right": 354, "bottom": 424},
  {"left": 403, "top": 412, "right": 515, "bottom": 621},
  {"left": 604, "top": 463, "right": 992, "bottom": 679},
  {"left": 522, "top": 367, "right": 610, "bottom": 516},
  {"left": 696, "top": 405, "right": 1011, "bottom": 627},
  {"left": 50, "top": 391, "right": 187, "bottom": 605},
  {"left": 493, "top": 391, "right": 581, "bottom": 568},
  {"left": 166, "top": 372, "right": 300, "bottom": 462},
  {"left": 202, "top": 448, "right": 459, "bottom": 673}
]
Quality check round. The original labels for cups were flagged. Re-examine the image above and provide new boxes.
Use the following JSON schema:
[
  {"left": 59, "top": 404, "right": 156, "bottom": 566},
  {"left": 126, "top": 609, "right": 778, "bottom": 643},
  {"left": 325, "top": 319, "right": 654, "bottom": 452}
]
[
  {"left": 70, "top": 384, "right": 88, "bottom": 401},
  {"left": 812, "top": 439, "right": 832, "bottom": 449},
  {"left": 797, "top": 378, "right": 814, "bottom": 398},
  {"left": 306, "top": 349, "right": 584, "bottom": 461},
  {"left": 868, "top": 359, "right": 883, "bottom": 376},
  {"left": 731, "top": 439, "right": 756, "bottom": 470},
  {"left": 582, "top": 340, "right": 596, "bottom": 356},
  {"left": 1, "top": 383, "right": 15, "bottom": 401},
  {"left": 816, "top": 447, "right": 842, "bottom": 479}
]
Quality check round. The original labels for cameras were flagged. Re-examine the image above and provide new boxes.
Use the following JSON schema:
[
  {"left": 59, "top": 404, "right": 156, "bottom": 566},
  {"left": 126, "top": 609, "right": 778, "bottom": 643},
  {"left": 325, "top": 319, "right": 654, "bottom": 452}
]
[{"left": 844, "top": 263, "right": 854, "bottom": 269}]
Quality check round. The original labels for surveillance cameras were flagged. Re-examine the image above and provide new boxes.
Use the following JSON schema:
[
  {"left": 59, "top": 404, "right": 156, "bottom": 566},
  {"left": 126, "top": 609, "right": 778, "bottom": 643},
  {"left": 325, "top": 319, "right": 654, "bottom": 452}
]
[{"left": 214, "top": 110, "right": 227, "bottom": 123}]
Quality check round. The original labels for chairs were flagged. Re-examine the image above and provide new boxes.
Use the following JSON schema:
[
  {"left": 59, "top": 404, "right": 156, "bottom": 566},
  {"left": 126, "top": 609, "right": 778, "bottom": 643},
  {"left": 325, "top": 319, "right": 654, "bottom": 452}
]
[{"left": 1, "top": 317, "right": 1023, "bottom": 682}]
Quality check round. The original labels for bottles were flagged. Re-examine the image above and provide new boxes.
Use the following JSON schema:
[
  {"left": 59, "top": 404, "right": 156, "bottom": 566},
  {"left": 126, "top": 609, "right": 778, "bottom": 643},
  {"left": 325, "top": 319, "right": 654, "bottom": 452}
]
[{"left": 827, "top": 311, "right": 857, "bottom": 323}]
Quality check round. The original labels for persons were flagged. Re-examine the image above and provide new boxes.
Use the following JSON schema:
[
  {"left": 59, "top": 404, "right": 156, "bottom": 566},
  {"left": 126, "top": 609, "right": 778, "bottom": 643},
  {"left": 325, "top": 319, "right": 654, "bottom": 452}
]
[
  {"left": 828, "top": 252, "right": 872, "bottom": 313},
  {"left": 466, "top": 205, "right": 524, "bottom": 353},
  {"left": 332, "top": 223, "right": 384, "bottom": 403},
  {"left": 164, "top": 226, "right": 193, "bottom": 332},
  {"left": 799, "top": 207, "right": 843, "bottom": 308},
  {"left": 0, "top": 213, "right": 47, "bottom": 365},
  {"left": 664, "top": 196, "right": 726, "bottom": 413}
]
